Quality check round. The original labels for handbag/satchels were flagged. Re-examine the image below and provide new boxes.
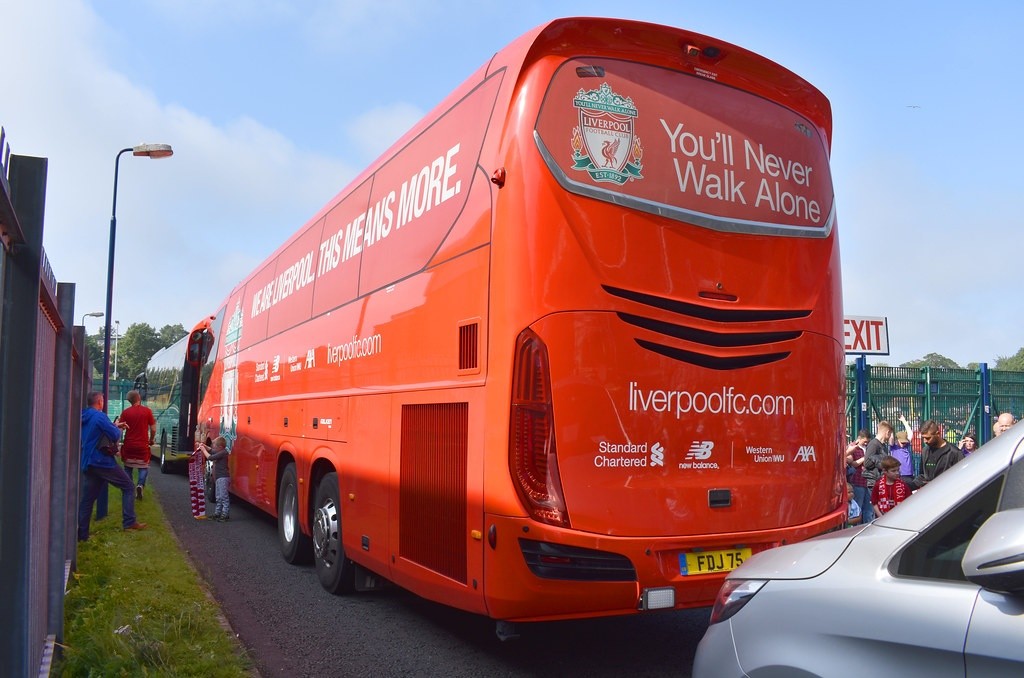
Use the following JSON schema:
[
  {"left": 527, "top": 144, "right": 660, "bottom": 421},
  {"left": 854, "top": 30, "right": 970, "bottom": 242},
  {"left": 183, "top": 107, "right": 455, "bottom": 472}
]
[{"left": 98, "top": 434, "right": 118, "bottom": 457}]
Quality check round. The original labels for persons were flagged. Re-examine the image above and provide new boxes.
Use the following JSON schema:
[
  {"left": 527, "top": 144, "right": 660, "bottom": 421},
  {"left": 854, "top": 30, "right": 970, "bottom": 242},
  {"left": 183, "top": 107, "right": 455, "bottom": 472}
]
[
  {"left": 847, "top": 482, "right": 862, "bottom": 528},
  {"left": 916, "top": 420, "right": 965, "bottom": 483},
  {"left": 869, "top": 456, "right": 910, "bottom": 517},
  {"left": 889, "top": 415, "right": 913, "bottom": 483},
  {"left": 957, "top": 434, "right": 978, "bottom": 456},
  {"left": 943, "top": 421, "right": 956, "bottom": 444},
  {"left": 911, "top": 430, "right": 922, "bottom": 476},
  {"left": 861, "top": 421, "right": 893, "bottom": 523},
  {"left": 77, "top": 391, "right": 146, "bottom": 541},
  {"left": 199, "top": 437, "right": 230, "bottom": 522},
  {"left": 993, "top": 413, "right": 1014, "bottom": 437},
  {"left": 118, "top": 390, "right": 156, "bottom": 500},
  {"left": 845, "top": 429, "right": 871, "bottom": 508}
]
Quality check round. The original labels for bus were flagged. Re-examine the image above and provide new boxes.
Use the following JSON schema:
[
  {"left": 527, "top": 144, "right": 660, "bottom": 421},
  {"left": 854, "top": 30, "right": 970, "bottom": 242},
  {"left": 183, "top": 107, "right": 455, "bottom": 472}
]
[
  {"left": 184, "top": 15, "right": 849, "bottom": 644},
  {"left": 132, "top": 334, "right": 199, "bottom": 474}
]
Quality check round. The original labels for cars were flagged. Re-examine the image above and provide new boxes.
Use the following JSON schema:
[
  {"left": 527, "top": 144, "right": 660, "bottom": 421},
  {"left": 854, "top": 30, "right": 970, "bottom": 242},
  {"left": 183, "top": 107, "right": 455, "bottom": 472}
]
[{"left": 692, "top": 418, "right": 1024, "bottom": 678}]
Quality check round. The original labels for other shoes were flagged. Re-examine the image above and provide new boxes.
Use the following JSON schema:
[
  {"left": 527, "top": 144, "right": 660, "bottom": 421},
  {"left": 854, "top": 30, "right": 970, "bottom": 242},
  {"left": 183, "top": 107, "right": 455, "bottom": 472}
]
[
  {"left": 125, "top": 519, "right": 149, "bottom": 532},
  {"left": 136, "top": 484, "right": 144, "bottom": 502},
  {"left": 217, "top": 514, "right": 231, "bottom": 522},
  {"left": 207, "top": 511, "right": 221, "bottom": 520}
]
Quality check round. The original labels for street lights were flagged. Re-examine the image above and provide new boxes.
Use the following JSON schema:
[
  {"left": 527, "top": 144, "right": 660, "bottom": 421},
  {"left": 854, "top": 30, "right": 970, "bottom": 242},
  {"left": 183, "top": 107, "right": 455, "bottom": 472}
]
[
  {"left": 102, "top": 143, "right": 173, "bottom": 417},
  {"left": 114, "top": 320, "right": 120, "bottom": 381}
]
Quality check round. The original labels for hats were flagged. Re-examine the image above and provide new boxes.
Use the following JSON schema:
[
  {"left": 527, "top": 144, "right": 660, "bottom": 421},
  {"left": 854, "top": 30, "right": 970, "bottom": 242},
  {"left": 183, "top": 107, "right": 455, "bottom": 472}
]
[{"left": 895, "top": 431, "right": 909, "bottom": 442}]
